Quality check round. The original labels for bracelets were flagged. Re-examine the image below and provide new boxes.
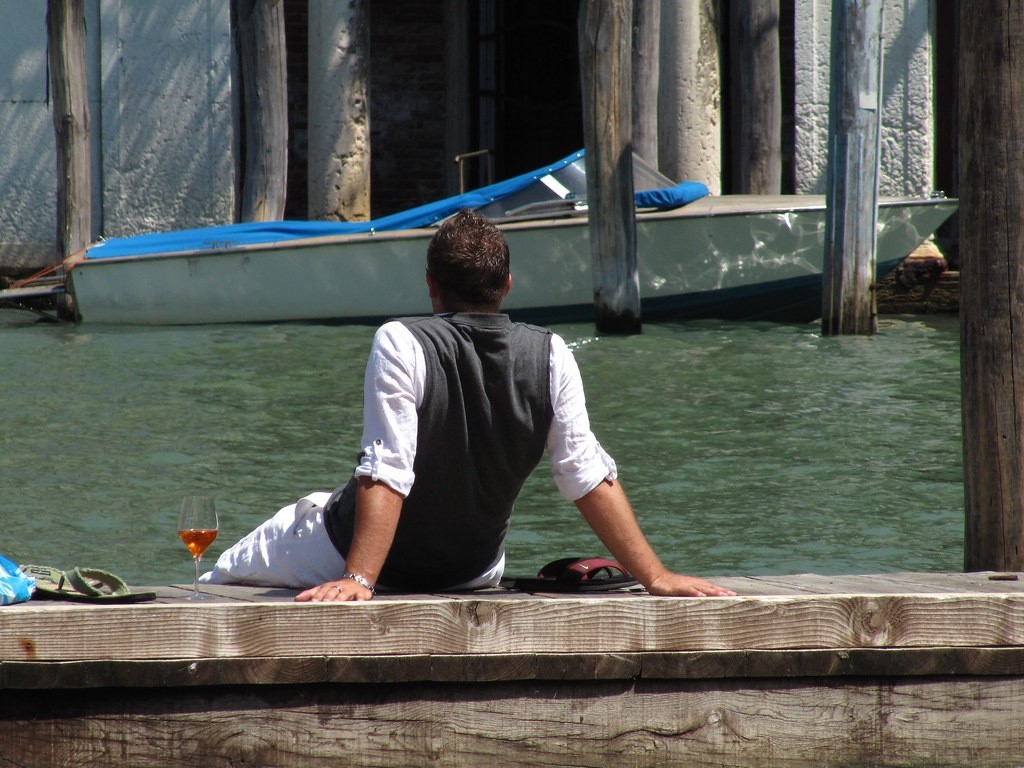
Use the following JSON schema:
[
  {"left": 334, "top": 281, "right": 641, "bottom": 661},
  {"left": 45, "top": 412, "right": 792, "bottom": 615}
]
[{"left": 342, "top": 573, "right": 375, "bottom": 595}]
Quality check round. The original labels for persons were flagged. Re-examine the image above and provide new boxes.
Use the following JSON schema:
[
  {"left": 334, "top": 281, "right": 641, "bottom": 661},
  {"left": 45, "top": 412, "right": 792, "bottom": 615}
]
[{"left": 199, "top": 209, "right": 736, "bottom": 602}]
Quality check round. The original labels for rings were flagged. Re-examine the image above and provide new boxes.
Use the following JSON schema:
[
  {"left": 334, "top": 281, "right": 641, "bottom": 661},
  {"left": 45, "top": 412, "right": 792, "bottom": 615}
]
[{"left": 332, "top": 585, "right": 341, "bottom": 593}]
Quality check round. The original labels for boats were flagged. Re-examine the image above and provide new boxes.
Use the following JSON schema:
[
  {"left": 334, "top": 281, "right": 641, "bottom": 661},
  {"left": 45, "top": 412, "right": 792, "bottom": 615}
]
[{"left": 58, "top": 139, "right": 959, "bottom": 327}]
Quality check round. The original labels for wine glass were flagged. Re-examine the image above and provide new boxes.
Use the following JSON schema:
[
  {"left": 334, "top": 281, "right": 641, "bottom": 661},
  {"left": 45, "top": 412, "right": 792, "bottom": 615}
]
[{"left": 177, "top": 495, "right": 219, "bottom": 601}]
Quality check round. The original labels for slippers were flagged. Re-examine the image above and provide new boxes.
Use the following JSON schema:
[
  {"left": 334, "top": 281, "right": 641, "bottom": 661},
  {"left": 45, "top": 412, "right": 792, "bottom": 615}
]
[
  {"left": 19, "top": 564, "right": 156, "bottom": 604},
  {"left": 498, "top": 557, "right": 640, "bottom": 592}
]
